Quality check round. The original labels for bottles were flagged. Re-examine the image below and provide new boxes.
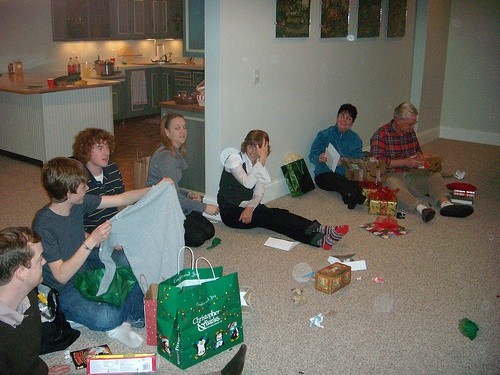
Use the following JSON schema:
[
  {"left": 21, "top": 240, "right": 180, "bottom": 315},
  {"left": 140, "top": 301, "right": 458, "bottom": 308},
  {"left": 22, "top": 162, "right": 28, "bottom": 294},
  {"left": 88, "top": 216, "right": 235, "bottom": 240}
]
[
  {"left": 68, "top": 58, "right": 75, "bottom": 75},
  {"left": 74, "top": 57, "right": 80, "bottom": 74},
  {"left": 110, "top": 49, "right": 116, "bottom": 72},
  {"left": 122, "top": 48, "right": 127, "bottom": 65}
]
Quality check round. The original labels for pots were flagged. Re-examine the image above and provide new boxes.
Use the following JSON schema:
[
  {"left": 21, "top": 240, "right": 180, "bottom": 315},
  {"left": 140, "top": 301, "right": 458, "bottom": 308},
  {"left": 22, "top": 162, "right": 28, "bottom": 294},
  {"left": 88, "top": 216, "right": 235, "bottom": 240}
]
[{"left": 93, "top": 60, "right": 114, "bottom": 76}]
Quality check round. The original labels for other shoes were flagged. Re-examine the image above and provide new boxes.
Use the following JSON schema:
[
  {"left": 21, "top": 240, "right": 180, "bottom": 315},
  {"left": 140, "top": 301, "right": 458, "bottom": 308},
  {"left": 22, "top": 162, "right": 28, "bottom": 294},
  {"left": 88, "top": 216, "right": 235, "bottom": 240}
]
[
  {"left": 440, "top": 204, "right": 474, "bottom": 218},
  {"left": 421, "top": 208, "right": 435, "bottom": 222},
  {"left": 346, "top": 187, "right": 367, "bottom": 210},
  {"left": 221, "top": 344, "right": 247, "bottom": 375}
]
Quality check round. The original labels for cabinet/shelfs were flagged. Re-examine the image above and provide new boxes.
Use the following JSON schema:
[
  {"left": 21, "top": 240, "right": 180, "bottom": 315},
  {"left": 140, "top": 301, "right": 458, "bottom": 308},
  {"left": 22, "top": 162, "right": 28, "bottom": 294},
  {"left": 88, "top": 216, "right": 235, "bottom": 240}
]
[
  {"left": 169, "top": 0, "right": 184, "bottom": 40},
  {"left": 125, "top": 68, "right": 162, "bottom": 118},
  {"left": 51, "top": 0, "right": 111, "bottom": 42},
  {"left": 171, "top": 68, "right": 205, "bottom": 100},
  {"left": 113, "top": 83, "right": 125, "bottom": 120},
  {"left": 183, "top": 0, "right": 205, "bottom": 58},
  {"left": 110, "top": 0, "right": 151, "bottom": 41},
  {"left": 160, "top": 67, "right": 174, "bottom": 102},
  {"left": 148, "top": 0, "right": 174, "bottom": 40}
]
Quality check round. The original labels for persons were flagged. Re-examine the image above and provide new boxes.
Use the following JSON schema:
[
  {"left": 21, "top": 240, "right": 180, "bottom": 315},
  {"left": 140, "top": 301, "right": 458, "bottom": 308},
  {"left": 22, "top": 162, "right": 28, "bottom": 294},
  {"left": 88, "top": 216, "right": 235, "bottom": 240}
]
[
  {"left": 370, "top": 102, "right": 474, "bottom": 223},
  {"left": 68, "top": 128, "right": 130, "bottom": 268},
  {"left": 33, "top": 156, "right": 174, "bottom": 333},
  {"left": 146, "top": 113, "right": 219, "bottom": 249},
  {"left": 308, "top": 103, "right": 367, "bottom": 209},
  {"left": 0, "top": 227, "right": 71, "bottom": 375},
  {"left": 217, "top": 129, "right": 349, "bottom": 250}
]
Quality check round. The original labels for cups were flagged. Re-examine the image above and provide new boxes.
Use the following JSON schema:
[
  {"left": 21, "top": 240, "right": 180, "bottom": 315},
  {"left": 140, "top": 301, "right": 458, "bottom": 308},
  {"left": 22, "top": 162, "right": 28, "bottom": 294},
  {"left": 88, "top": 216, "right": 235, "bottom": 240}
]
[{"left": 48, "top": 79, "right": 54, "bottom": 88}]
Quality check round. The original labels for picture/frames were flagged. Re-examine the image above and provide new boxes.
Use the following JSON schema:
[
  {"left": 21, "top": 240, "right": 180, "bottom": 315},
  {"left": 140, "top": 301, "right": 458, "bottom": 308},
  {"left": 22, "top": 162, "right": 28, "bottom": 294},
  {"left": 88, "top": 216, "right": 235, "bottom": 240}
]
[
  {"left": 274, "top": 0, "right": 311, "bottom": 41},
  {"left": 354, "top": 0, "right": 384, "bottom": 41},
  {"left": 318, "top": 0, "right": 351, "bottom": 41},
  {"left": 384, "top": 0, "right": 410, "bottom": 41}
]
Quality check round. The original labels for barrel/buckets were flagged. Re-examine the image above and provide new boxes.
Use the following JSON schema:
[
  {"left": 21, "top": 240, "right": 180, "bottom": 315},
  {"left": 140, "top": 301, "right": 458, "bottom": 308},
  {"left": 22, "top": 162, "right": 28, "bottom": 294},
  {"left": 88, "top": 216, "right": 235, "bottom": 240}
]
[{"left": 133, "top": 157, "right": 151, "bottom": 190}]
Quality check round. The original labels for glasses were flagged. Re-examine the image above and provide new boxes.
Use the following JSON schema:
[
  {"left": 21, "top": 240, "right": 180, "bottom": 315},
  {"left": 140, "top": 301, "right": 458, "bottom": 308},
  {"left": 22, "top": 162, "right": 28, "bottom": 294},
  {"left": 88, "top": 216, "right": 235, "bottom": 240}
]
[{"left": 402, "top": 118, "right": 417, "bottom": 126}]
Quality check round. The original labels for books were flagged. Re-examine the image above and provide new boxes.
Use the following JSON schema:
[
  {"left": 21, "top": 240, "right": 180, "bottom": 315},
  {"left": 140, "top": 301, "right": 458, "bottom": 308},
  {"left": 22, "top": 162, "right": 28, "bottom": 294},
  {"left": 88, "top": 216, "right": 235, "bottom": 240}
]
[{"left": 70, "top": 344, "right": 112, "bottom": 370}]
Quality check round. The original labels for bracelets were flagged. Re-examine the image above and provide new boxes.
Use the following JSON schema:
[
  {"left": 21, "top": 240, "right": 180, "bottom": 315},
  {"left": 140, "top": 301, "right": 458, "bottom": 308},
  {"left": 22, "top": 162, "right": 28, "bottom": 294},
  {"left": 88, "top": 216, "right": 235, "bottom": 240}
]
[{"left": 83, "top": 243, "right": 92, "bottom": 251}]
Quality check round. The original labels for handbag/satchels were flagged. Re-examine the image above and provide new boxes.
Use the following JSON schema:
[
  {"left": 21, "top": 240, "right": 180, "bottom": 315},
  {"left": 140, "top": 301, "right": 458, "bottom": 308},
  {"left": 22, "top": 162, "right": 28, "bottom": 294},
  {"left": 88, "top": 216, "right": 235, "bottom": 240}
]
[
  {"left": 39, "top": 310, "right": 81, "bottom": 356},
  {"left": 280, "top": 158, "right": 315, "bottom": 198},
  {"left": 157, "top": 246, "right": 245, "bottom": 371},
  {"left": 139, "top": 273, "right": 158, "bottom": 347},
  {"left": 72, "top": 265, "right": 136, "bottom": 306},
  {"left": 38, "top": 284, "right": 59, "bottom": 323}
]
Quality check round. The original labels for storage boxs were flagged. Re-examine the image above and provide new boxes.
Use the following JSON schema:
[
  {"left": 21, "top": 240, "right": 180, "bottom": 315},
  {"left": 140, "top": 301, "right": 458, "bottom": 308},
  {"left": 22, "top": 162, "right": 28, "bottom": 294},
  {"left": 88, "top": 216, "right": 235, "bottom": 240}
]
[
  {"left": 367, "top": 196, "right": 398, "bottom": 218},
  {"left": 314, "top": 262, "right": 352, "bottom": 295},
  {"left": 342, "top": 157, "right": 387, "bottom": 183},
  {"left": 86, "top": 353, "right": 156, "bottom": 375},
  {"left": 362, "top": 188, "right": 381, "bottom": 205}
]
